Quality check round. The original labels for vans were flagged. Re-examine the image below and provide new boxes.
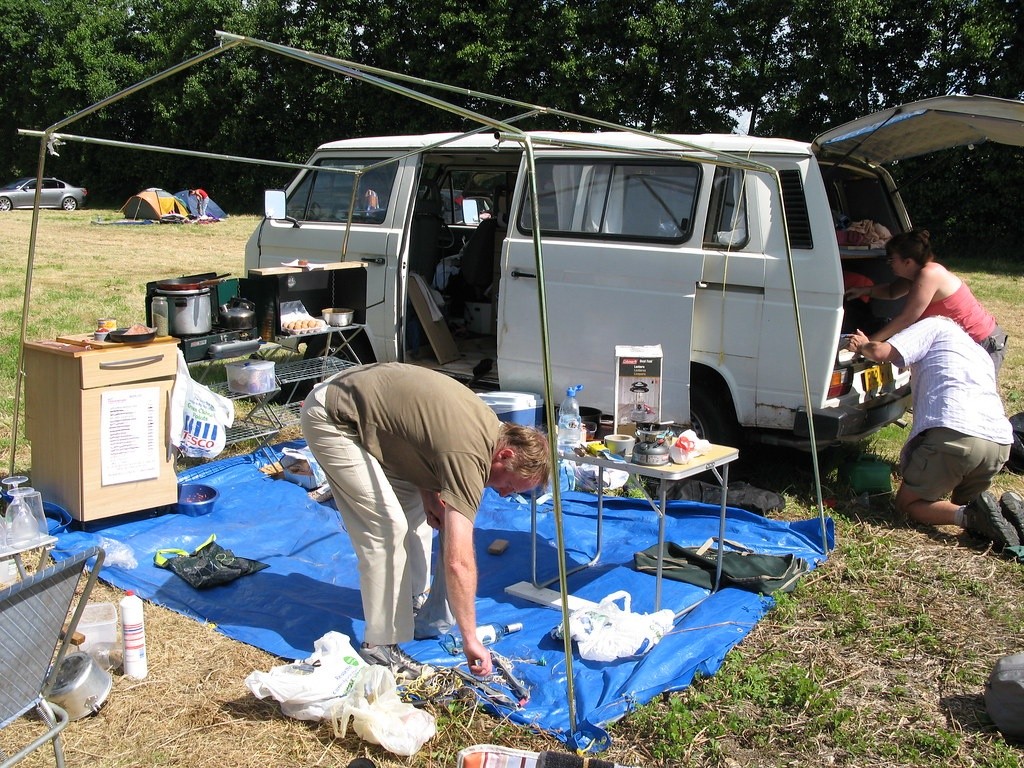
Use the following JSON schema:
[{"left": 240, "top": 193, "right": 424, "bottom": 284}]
[{"left": 244, "top": 94, "right": 1024, "bottom": 478}]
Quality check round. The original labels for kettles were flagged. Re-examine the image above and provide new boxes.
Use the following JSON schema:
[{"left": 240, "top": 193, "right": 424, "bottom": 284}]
[{"left": 218, "top": 297, "right": 256, "bottom": 329}]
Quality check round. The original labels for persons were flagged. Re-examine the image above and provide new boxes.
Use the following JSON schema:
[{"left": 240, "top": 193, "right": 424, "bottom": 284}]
[
  {"left": 844, "top": 315, "right": 1024, "bottom": 550},
  {"left": 846, "top": 230, "right": 1010, "bottom": 377},
  {"left": 188, "top": 188, "right": 210, "bottom": 215},
  {"left": 301, "top": 362, "right": 550, "bottom": 685}
]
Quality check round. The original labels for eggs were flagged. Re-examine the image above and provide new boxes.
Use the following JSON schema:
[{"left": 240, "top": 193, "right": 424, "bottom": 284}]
[{"left": 283, "top": 319, "right": 321, "bottom": 329}]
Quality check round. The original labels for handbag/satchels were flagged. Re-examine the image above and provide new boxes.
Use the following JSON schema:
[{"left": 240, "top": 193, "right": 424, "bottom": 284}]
[
  {"left": 553, "top": 590, "right": 674, "bottom": 664},
  {"left": 168, "top": 349, "right": 234, "bottom": 458},
  {"left": 154, "top": 533, "right": 271, "bottom": 591},
  {"left": 635, "top": 536, "right": 810, "bottom": 597},
  {"left": 243, "top": 631, "right": 437, "bottom": 756}
]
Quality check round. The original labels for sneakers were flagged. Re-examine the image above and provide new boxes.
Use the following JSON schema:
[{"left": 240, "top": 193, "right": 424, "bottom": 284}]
[
  {"left": 997, "top": 490, "right": 1024, "bottom": 540},
  {"left": 357, "top": 641, "right": 424, "bottom": 680},
  {"left": 963, "top": 491, "right": 1019, "bottom": 551},
  {"left": 412, "top": 589, "right": 432, "bottom": 618}
]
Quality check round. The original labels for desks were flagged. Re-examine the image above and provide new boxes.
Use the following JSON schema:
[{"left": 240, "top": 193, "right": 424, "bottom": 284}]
[{"left": 530, "top": 434, "right": 739, "bottom": 620}]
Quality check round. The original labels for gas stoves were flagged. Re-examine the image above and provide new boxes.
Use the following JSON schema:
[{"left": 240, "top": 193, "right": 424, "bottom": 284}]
[{"left": 176, "top": 322, "right": 258, "bottom": 363}]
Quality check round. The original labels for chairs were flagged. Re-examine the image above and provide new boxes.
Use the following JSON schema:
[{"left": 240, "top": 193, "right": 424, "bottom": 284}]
[{"left": 0, "top": 545, "right": 106, "bottom": 761}]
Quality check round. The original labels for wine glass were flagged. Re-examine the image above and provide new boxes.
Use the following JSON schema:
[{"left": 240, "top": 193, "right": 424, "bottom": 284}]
[{"left": 2, "top": 475, "right": 41, "bottom": 548}]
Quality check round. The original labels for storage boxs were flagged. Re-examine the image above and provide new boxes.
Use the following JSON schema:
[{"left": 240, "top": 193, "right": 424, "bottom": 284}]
[
  {"left": 73, "top": 602, "right": 118, "bottom": 655},
  {"left": 477, "top": 391, "right": 544, "bottom": 428},
  {"left": 464, "top": 302, "right": 491, "bottom": 335}
]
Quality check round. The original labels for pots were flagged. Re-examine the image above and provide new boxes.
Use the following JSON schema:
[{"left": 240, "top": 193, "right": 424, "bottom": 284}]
[
  {"left": 42, "top": 651, "right": 111, "bottom": 721},
  {"left": 156, "top": 273, "right": 226, "bottom": 335}
]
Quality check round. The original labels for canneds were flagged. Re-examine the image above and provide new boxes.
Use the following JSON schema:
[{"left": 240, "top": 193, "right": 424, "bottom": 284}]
[{"left": 98, "top": 317, "right": 116, "bottom": 332}]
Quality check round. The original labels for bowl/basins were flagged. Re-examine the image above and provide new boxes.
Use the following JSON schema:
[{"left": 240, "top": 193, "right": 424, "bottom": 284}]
[
  {"left": 94, "top": 330, "right": 110, "bottom": 341},
  {"left": 174, "top": 483, "right": 220, "bottom": 517},
  {"left": 322, "top": 307, "right": 354, "bottom": 326},
  {"left": 109, "top": 330, "right": 156, "bottom": 342},
  {"left": 579, "top": 405, "right": 602, "bottom": 440}
]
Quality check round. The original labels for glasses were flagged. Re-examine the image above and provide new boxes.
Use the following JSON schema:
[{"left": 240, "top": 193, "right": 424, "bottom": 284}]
[{"left": 887, "top": 256, "right": 904, "bottom": 264}]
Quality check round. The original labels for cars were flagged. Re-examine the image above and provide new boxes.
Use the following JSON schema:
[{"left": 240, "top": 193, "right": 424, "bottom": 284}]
[
  {"left": 369, "top": 190, "right": 464, "bottom": 224},
  {"left": 0, "top": 177, "right": 88, "bottom": 211}
]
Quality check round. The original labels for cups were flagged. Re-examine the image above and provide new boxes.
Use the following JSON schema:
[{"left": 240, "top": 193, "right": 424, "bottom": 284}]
[{"left": 25, "top": 489, "right": 47, "bottom": 539}]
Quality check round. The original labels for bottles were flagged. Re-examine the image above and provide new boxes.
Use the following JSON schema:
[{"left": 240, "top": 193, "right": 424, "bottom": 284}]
[
  {"left": 261, "top": 305, "right": 273, "bottom": 341},
  {"left": 444, "top": 623, "right": 524, "bottom": 655},
  {"left": 152, "top": 297, "right": 168, "bottom": 337},
  {"left": 557, "top": 384, "right": 581, "bottom": 456},
  {"left": 119, "top": 590, "right": 147, "bottom": 680}
]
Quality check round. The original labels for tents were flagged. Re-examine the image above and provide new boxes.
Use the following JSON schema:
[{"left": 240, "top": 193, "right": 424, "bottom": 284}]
[{"left": 123, "top": 188, "right": 227, "bottom": 221}]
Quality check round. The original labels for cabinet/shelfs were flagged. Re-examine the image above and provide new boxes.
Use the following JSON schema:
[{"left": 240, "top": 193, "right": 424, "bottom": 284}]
[{"left": 21, "top": 338, "right": 182, "bottom": 531}]
